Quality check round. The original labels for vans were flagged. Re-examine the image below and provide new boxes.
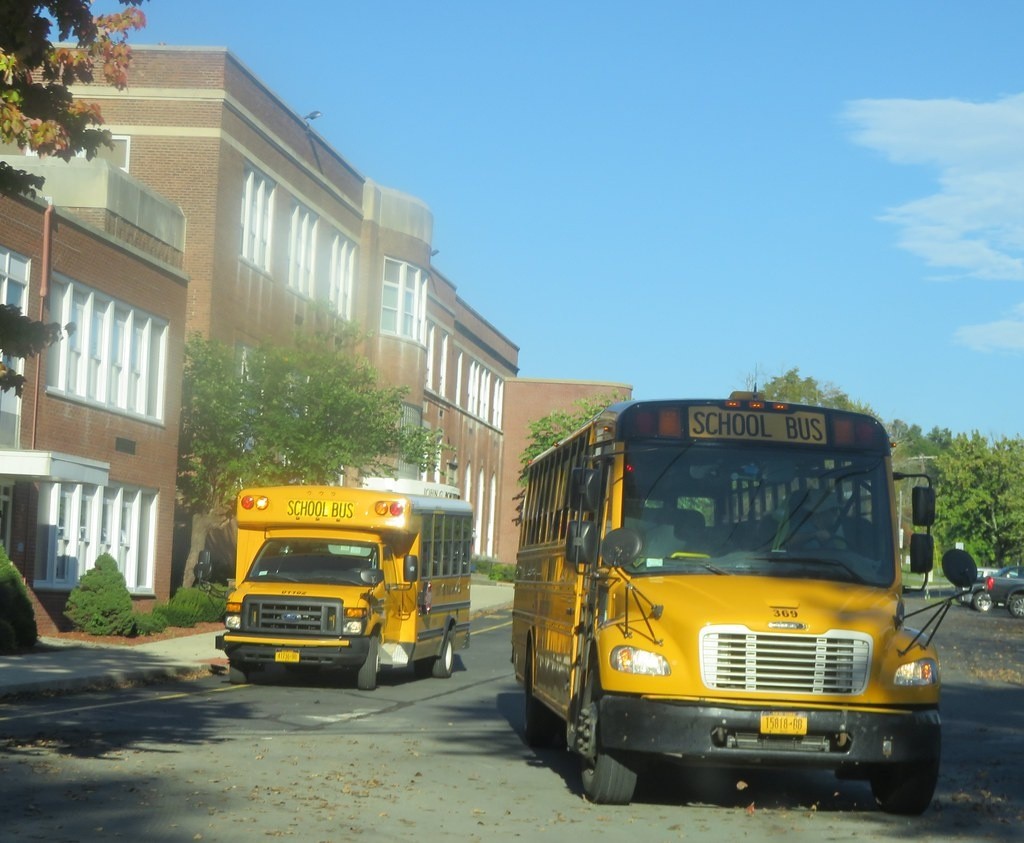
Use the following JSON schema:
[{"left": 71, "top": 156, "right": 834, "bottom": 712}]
[{"left": 977, "top": 568, "right": 1017, "bottom": 582}]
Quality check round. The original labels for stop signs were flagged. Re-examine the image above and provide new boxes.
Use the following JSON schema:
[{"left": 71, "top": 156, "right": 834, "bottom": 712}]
[{"left": 424, "top": 584, "right": 433, "bottom": 616}]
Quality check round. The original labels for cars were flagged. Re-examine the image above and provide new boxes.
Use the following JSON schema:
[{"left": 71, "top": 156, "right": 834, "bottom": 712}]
[{"left": 952, "top": 564, "right": 1024, "bottom": 617}]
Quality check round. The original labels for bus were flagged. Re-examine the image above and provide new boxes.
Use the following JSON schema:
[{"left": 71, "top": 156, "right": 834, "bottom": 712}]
[
  {"left": 507, "top": 388, "right": 977, "bottom": 805},
  {"left": 217, "top": 483, "right": 476, "bottom": 692}
]
